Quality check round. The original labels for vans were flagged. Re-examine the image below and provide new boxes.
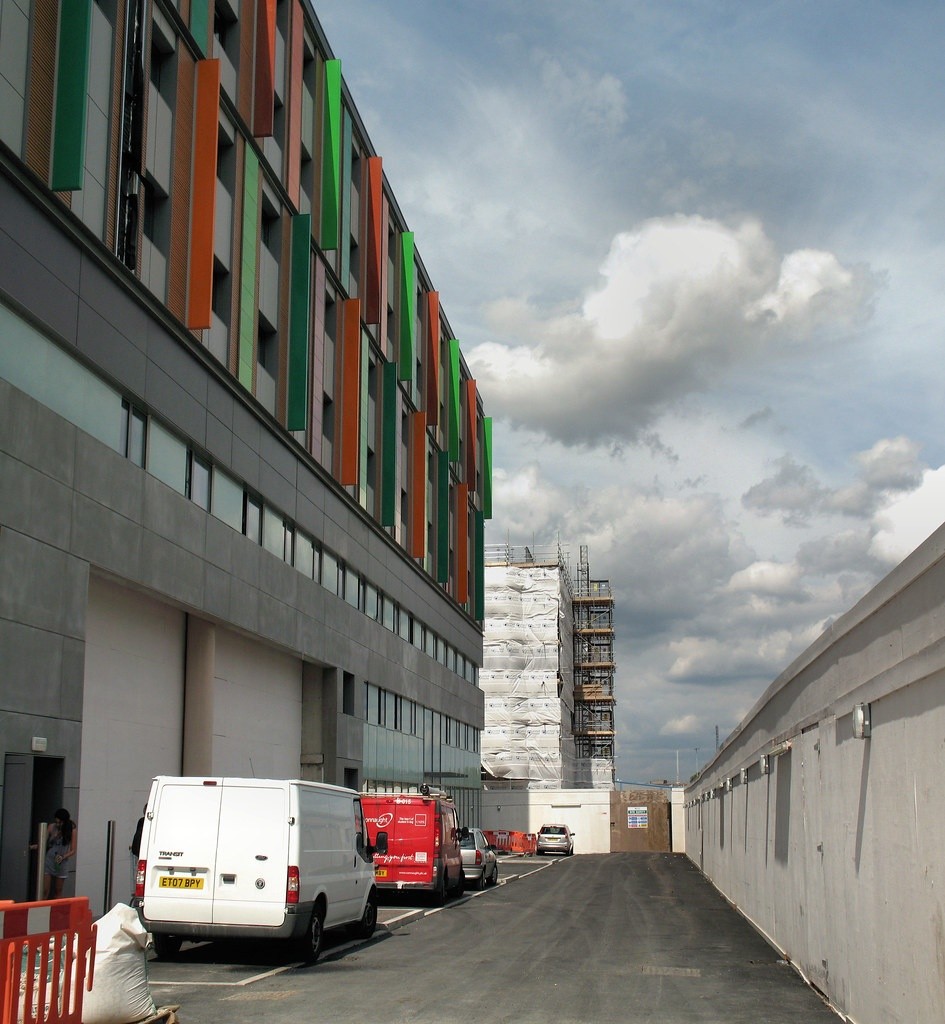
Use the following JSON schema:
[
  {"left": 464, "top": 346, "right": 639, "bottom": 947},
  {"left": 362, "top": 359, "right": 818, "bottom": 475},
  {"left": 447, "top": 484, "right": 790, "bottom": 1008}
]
[
  {"left": 135, "top": 775, "right": 388, "bottom": 964},
  {"left": 357, "top": 791, "right": 470, "bottom": 903}
]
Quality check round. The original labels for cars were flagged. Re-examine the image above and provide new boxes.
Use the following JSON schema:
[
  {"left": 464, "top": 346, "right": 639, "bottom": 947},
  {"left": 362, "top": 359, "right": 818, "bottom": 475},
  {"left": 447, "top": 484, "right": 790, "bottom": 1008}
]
[{"left": 458, "top": 827, "right": 499, "bottom": 890}]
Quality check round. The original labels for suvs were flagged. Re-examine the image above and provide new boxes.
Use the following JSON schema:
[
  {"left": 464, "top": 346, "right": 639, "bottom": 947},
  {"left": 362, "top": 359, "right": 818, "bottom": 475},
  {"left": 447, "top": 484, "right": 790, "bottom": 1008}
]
[{"left": 536, "top": 824, "right": 576, "bottom": 854}]
